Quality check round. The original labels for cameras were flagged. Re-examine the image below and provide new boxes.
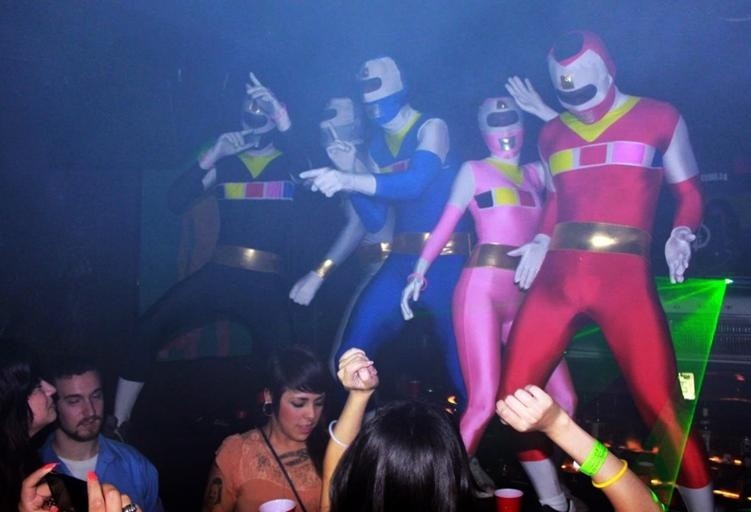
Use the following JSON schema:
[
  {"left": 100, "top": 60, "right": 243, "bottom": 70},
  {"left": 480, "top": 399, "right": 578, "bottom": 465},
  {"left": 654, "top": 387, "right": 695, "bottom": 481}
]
[{"left": 45, "top": 471, "right": 87, "bottom": 512}]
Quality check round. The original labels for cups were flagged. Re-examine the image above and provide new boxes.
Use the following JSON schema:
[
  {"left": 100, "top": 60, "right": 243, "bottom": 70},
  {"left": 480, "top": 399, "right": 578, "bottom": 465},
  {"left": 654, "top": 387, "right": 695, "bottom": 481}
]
[
  {"left": 259, "top": 498, "right": 297, "bottom": 512},
  {"left": 495, "top": 488, "right": 524, "bottom": 512}
]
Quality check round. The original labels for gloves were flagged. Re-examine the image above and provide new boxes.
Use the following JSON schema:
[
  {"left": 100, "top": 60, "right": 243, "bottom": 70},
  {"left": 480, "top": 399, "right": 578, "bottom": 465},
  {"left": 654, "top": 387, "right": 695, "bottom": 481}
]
[
  {"left": 663, "top": 226, "right": 697, "bottom": 285},
  {"left": 298, "top": 163, "right": 378, "bottom": 201},
  {"left": 244, "top": 72, "right": 290, "bottom": 131},
  {"left": 504, "top": 72, "right": 562, "bottom": 129},
  {"left": 197, "top": 128, "right": 256, "bottom": 171},
  {"left": 400, "top": 257, "right": 430, "bottom": 322},
  {"left": 288, "top": 270, "right": 323, "bottom": 308},
  {"left": 506, "top": 233, "right": 551, "bottom": 291},
  {"left": 325, "top": 122, "right": 358, "bottom": 173}
]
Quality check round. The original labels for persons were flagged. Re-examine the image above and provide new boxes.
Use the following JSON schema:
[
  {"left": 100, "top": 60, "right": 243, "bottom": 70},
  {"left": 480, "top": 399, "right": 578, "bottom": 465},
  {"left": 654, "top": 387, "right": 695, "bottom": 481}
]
[
  {"left": 494, "top": 31, "right": 714, "bottom": 511},
  {"left": 320, "top": 95, "right": 510, "bottom": 477},
  {"left": 321, "top": 348, "right": 668, "bottom": 511},
  {"left": 17, "top": 464, "right": 142, "bottom": 512},
  {"left": 288, "top": 98, "right": 405, "bottom": 386},
  {"left": 400, "top": 96, "right": 579, "bottom": 512},
  {"left": 35, "top": 364, "right": 161, "bottom": 512},
  {"left": 0, "top": 354, "right": 56, "bottom": 512},
  {"left": 113, "top": 71, "right": 339, "bottom": 436},
  {"left": 289, "top": 51, "right": 498, "bottom": 501},
  {"left": 203, "top": 346, "right": 323, "bottom": 512}
]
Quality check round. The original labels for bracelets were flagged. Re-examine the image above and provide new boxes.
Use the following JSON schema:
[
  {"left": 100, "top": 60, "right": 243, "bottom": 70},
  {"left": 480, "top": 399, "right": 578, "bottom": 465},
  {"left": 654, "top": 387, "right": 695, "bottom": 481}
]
[
  {"left": 579, "top": 440, "right": 608, "bottom": 477},
  {"left": 328, "top": 420, "right": 350, "bottom": 449},
  {"left": 592, "top": 460, "right": 628, "bottom": 490},
  {"left": 307, "top": 256, "right": 339, "bottom": 281},
  {"left": 407, "top": 274, "right": 428, "bottom": 291}
]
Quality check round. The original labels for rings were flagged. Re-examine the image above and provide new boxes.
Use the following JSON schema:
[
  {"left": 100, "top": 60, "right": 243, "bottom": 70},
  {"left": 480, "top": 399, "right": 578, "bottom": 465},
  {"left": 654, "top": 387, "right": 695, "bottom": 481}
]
[{"left": 122, "top": 504, "right": 137, "bottom": 512}]
